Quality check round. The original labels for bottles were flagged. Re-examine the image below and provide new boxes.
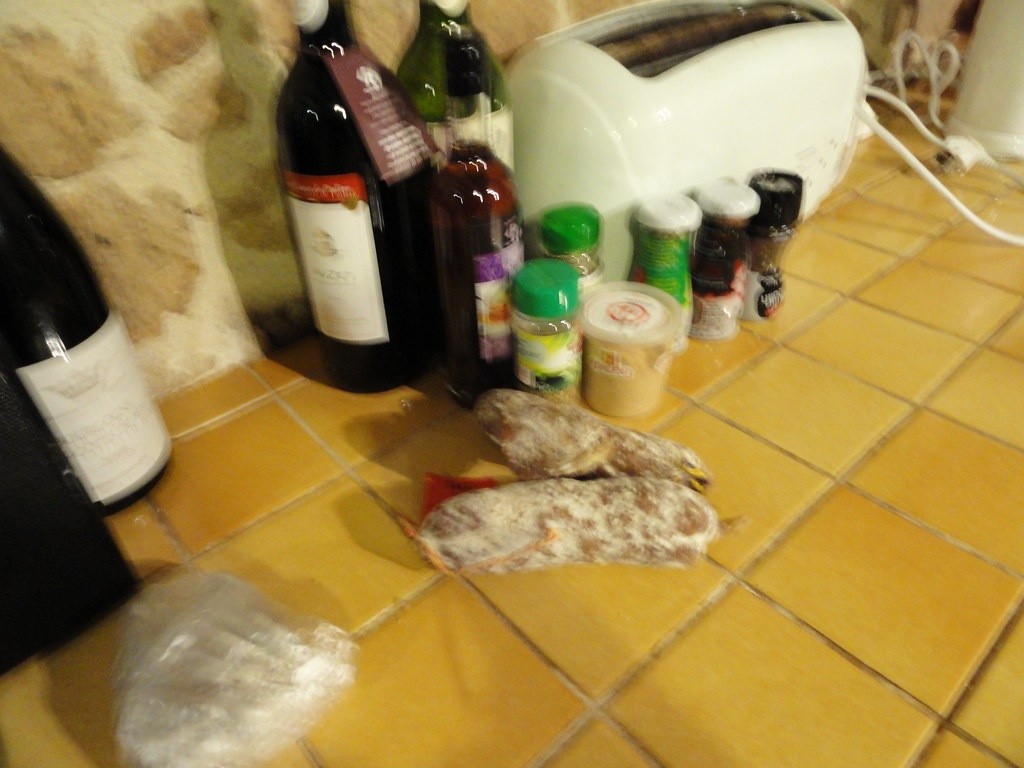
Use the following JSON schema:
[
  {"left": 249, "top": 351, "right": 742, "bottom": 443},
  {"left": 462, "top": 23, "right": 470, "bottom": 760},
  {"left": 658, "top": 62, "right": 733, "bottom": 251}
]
[
  {"left": 0, "top": 143, "right": 173, "bottom": 515},
  {"left": 275, "top": 1, "right": 803, "bottom": 409}
]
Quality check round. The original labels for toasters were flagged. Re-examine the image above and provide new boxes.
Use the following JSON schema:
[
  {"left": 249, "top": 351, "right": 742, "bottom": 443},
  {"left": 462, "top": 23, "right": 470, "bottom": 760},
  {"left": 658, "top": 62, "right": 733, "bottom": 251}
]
[{"left": 485, "top": 1, "right": 870, "bottom": 276}]
